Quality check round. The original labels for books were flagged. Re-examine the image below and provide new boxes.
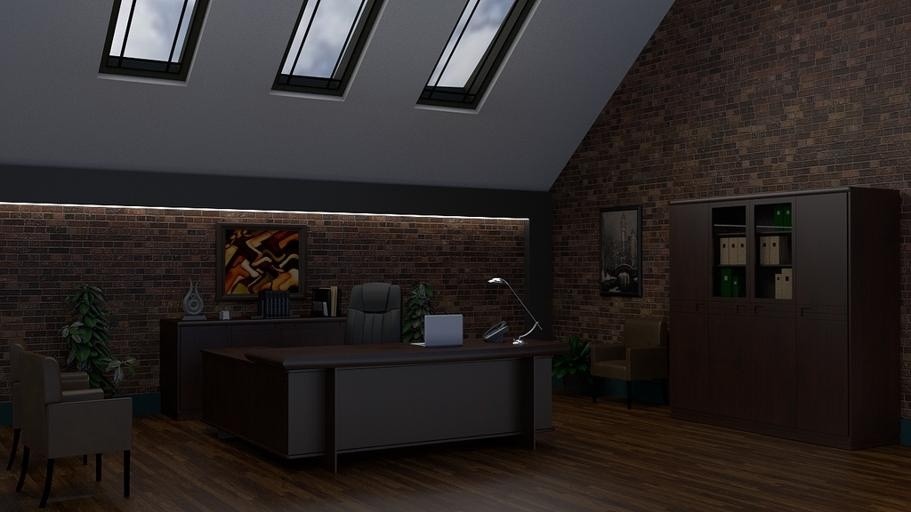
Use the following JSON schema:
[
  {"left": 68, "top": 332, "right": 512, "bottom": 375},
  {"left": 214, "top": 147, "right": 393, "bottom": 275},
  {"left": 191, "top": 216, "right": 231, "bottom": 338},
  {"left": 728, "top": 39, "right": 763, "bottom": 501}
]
[
  {"left": 254, "top": 290, "right": 291, "bottom": 319},
  {"left": 311, "top": 285, "right": 341, "bottom": 317}
]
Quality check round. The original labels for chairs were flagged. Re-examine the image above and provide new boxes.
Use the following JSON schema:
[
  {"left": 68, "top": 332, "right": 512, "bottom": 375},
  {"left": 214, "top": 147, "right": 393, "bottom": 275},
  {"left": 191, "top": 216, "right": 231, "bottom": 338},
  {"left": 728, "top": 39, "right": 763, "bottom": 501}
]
[
  {"left": 590, "top": 318, "right": 668, "bottom": 409},
  {"left": 345, "top": 282, "right": 403, "bottom": 345},
  {"left": 6, "top": 335, "right": 133, "bottom": 508}
]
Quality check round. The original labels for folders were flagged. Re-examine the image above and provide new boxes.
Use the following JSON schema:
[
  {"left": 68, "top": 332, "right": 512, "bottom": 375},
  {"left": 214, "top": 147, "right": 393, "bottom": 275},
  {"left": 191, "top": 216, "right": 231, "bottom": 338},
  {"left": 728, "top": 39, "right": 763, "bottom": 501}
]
[
  {"left": 720, "top": 237, "right": 746, "bottom": 264},
  {"left": 782, "top": 269, "right": 793, "bottom": 300},
  {"left": 775, "top": 274, "right": 782, "bottom": 299},
  {"left": 759, "top": 237, "right": 788, "bottom": 264},
  {"left": 719, "top": 267, "right": 741, "bottom": 297}
]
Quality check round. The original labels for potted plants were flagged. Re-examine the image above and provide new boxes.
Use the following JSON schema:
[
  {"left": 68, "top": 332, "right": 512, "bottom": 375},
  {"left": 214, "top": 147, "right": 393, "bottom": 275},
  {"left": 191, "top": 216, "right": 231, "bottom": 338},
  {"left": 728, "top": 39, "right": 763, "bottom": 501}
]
[{"left": 553, "top": 335, "right": 591, "bottom": 394}]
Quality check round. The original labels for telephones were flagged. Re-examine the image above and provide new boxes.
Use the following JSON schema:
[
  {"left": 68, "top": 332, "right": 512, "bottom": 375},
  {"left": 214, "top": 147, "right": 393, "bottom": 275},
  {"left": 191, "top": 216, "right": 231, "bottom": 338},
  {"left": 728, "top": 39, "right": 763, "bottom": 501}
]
[{"left": 483, "top": 320, "right": 509, "bottom": 342}]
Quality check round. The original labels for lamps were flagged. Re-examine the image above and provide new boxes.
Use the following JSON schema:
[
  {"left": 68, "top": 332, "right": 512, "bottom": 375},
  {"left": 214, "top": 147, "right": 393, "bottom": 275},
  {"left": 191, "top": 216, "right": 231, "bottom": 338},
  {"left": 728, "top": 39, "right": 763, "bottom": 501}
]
[{"left": 487, "top": 276, "right": 543, "bottom": 345}]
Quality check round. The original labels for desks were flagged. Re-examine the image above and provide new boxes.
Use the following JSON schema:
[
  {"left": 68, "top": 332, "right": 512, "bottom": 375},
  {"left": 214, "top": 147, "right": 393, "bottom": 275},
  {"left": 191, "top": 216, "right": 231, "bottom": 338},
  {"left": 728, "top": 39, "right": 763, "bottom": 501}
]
[{"left": 199, "top": 337, "right": 568, "bottom": 475}]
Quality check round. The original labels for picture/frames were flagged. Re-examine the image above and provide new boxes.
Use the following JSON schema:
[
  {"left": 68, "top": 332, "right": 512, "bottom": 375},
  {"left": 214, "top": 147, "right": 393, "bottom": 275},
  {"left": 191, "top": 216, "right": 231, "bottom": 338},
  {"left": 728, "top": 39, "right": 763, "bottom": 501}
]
[
  {"left": 598, "top": 203, "right": 643, "bottom": 298},
  {"left": 216, "top": 222, "right": 308, "bottom": 302}
]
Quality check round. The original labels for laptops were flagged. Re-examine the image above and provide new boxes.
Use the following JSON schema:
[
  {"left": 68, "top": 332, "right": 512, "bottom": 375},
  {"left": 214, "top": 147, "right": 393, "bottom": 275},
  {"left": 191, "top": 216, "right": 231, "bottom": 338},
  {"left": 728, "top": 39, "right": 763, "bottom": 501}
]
[{"left": 408, "top": 314, "right": 463, "bottom": 348}]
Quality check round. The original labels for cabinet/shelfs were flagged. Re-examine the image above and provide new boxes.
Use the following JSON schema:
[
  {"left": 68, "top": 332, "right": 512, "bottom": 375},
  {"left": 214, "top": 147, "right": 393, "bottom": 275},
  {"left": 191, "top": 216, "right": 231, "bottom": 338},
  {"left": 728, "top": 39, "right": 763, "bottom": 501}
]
[
  {"left": 159, "top": 315, "right": 347, "bottom": 422},
  {"left": 669, "top": 186, "right": 900, "bottom": 450}
]
